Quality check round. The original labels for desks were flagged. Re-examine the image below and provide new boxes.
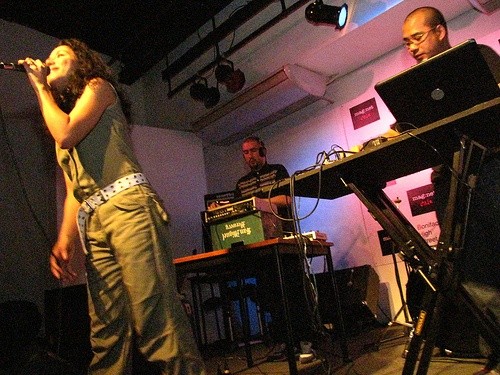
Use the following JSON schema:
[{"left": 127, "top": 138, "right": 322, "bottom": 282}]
[
  {"left": 172, "top": 237, "right": 349, "bottom": 374},
  {"left": 252, "top": 95, "right": 500, "bottom": 375}
]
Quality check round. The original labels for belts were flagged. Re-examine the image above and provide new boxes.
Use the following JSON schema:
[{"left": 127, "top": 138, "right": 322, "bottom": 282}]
[{"left": 76, "top": 173, "right": 148, "bottom": 255}]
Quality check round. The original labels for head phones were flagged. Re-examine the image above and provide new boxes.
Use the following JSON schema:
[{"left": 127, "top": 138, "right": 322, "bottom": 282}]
[{"left": 259, "top": 141, "right": 267, "bottom": 157}]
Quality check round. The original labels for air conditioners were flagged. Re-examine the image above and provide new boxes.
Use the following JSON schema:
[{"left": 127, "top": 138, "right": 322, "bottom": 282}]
[{"left": 191, "top": 64, "right": 335, "bottom": 147}]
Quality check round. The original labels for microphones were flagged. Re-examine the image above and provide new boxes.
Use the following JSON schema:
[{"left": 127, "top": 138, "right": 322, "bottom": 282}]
[{"left": 0, "top": 62, "right": 50, "bottom": 76}]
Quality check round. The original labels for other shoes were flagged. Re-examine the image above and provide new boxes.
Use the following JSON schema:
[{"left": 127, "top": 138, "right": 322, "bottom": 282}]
[
  {"left": 299, "top": 341, "right": 315, "bottom": 362},
  {"left": 266, "top": 343, "right": 298, "bottom": 362}
]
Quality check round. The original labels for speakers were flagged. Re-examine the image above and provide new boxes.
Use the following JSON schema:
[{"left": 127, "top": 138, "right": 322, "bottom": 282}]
[{"left": 315, "top": 264, "right": 380, "bottom": 326}]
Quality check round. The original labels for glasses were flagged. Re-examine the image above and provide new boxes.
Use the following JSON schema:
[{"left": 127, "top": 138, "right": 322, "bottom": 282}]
[{"left": 401, "top": 26, "right": 435, "bottom": 49}]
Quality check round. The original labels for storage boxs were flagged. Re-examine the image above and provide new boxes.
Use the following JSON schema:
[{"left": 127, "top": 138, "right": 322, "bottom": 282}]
[{"left": 210, "top": 211, "right": 283, "bottom": 251}]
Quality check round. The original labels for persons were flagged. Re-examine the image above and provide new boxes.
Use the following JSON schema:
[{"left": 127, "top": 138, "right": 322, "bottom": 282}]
[
  {"left": 208, "top": 137, "right": 316, "bottom": 363},
  {"left": 18, "top": 39, "right": 205, "bottom": 375},
  {"left": 354, "top": 7, "right": 500, "bottom": 375}
]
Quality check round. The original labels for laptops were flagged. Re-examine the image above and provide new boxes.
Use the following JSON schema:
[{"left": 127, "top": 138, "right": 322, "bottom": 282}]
[{"left": 375, "top": 38, "right": 500, "bottom": 131}]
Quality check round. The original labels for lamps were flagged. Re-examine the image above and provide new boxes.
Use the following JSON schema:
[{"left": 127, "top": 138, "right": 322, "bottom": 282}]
[
  {"left": 215, "top": 59, "right": 246, "bottom": 94},
  {"left": 190, "top": 78, "right": 220, "bottom": 109},
  {"left": 305, "top": 0, "right": 348, "bottom": 29}
]
